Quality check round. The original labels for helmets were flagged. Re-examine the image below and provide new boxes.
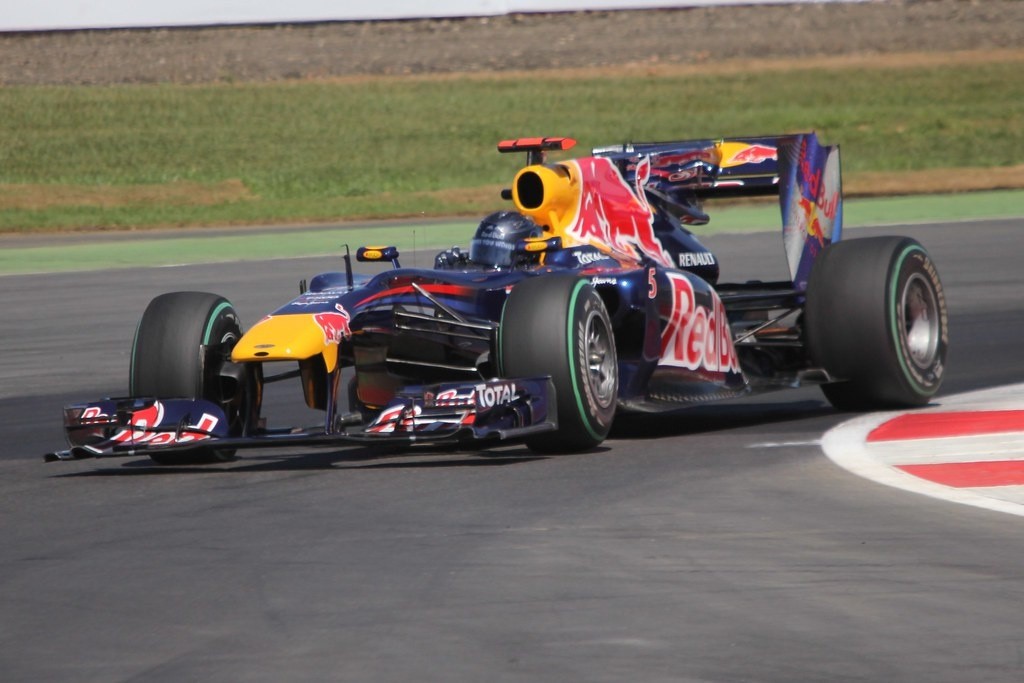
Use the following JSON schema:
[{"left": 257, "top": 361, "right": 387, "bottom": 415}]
[{"left": 468, "top": 209, "right": 543, "bottom": 271}]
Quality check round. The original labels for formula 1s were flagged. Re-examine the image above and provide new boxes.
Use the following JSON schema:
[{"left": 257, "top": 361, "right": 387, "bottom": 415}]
[{"left": 41, "top": 130, "right": 952, "bottom": 465}]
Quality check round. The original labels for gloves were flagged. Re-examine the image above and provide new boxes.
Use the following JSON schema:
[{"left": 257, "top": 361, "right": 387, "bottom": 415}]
[{"left": 434, "top": 246, "right": 473, "bottom": 272}]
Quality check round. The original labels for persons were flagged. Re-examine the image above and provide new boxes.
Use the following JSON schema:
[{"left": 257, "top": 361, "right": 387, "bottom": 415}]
[{"left": 433, "top": 209, "right": 546, "bottom": 272}]
